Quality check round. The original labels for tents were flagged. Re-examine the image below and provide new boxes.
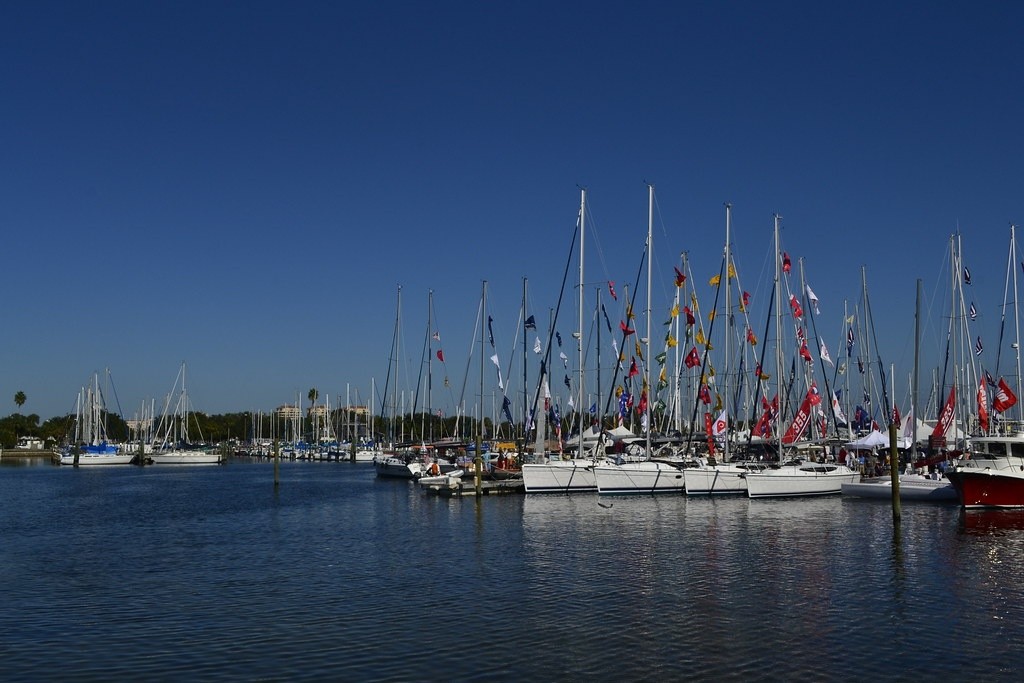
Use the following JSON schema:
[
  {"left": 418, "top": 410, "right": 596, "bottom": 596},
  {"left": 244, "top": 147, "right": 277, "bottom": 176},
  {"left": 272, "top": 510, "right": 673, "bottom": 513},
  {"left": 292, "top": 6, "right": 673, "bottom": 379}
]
[{"left": 845, "top": 408, "right": 970, "bottom": 460}]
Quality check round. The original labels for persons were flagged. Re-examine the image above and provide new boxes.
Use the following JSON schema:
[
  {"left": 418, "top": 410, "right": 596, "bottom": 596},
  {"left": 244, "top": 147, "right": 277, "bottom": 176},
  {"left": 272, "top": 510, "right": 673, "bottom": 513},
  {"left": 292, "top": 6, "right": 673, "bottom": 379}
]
[
  {"left": 841, "top": 447, "right": 945, "bottom": 479},
  {"left": 497, "top": 447, "right": 512, "bottom": 470},
  {"left": 992, "top": 416, "right": 1000, "bottom": 436},
  {"left": 600, "top": 428, "right": 607, "bottom": 458},
  {"left": 432, "top": 460, "right": 442, "bottom": 476},
  {"left": 614, "top": 438, "right": 623, "bottom": 465}
]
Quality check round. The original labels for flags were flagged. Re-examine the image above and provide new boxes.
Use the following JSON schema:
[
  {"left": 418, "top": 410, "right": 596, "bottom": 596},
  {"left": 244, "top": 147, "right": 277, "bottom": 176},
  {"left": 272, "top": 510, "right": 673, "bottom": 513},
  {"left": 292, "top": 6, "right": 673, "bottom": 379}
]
[
  {"left": 903, "top": 405, "right": 915, "bottom": 437},
  {"left": 991, "top": 378, "right": 1018, "bottom": 414},
  {"left": 892, "top": 402, "right": 901, "bottom": 430},
  {"left": 930, "top": 382, "right": 955, "bottom": 447},
  {"left": 985, "top": 370, "right": 997, "bottom": 388},
  {"left": 433, "top": 249, "right": 881, "bottom": 457},
  {"left": 977, "top": 375, "right": 988, "bottom": 433},
  {"left": 964, "top": 267, "right": 983, "bottom": 356}
]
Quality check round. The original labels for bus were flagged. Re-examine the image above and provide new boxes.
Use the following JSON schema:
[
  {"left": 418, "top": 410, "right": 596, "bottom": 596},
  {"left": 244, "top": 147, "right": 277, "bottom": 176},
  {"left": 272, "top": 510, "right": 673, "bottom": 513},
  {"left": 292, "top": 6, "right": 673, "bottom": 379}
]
[{"left": 14, "top": 436, "right": 41, "bottom": 449}]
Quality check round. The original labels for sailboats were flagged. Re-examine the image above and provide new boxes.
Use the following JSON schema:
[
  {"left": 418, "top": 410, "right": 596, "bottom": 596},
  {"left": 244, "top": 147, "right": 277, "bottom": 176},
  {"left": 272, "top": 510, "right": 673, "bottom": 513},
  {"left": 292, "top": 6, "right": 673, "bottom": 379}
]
[
  {"left": 236, "top": 382, "right": 371, "bottom": 462},
  {"left": 373, "top": 184, "right": 1024, "bottom": 510},
  {"left": 53, "top": 361, "right": 224, "bottom": 464}
]
